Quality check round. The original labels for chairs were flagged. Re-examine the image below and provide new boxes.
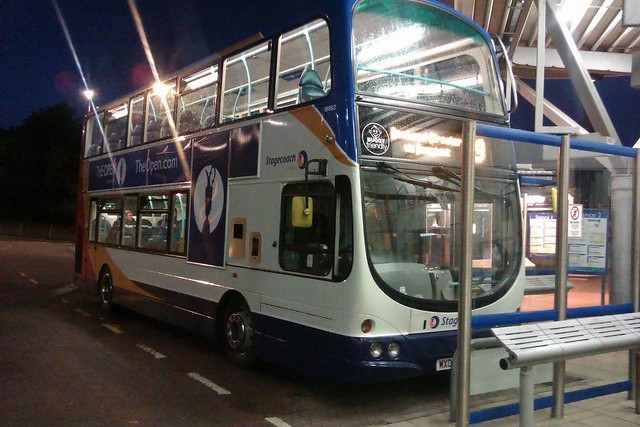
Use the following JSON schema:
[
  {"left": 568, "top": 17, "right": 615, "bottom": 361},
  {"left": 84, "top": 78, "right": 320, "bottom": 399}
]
[
  {"left": 119, "top": 129, "right": 127, "bottom": 151},
  {"left": 97, "top": 140, "right": 103, "bottom": 155},
  {"left": 147, "top": 122, "right": 160, "bottom": 142},
  {"left": 133, "top": 126, "right": 143, "bottom": 146},
  {"left": 161, "top": 116, "right": 174, "bottom": 139},
  {"left": 297, "top": 69, "right": 326, "bottom": 104},
  {"left": 87, "top": 143, "right": 98, "bottom": 157},
  {"left": 108, "top": 133, "right": 118, "bottom": 153},
  {"left": 206, "top": 116, "right": 217, "bottom": 127},
  {"left": 180, "top": 110, "right": 200, "bottom": 130}
]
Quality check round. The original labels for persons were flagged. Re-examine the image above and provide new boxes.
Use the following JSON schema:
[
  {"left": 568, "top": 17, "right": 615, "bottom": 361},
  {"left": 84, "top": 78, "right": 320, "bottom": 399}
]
[{"left": 115, "top": 208, "right": 136, "bottom": 226}]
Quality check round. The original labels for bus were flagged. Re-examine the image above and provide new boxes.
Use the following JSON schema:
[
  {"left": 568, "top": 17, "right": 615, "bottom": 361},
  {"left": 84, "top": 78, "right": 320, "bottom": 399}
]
[{"left": 75, "top": 0, "right": 526, "bottom": 386}]
